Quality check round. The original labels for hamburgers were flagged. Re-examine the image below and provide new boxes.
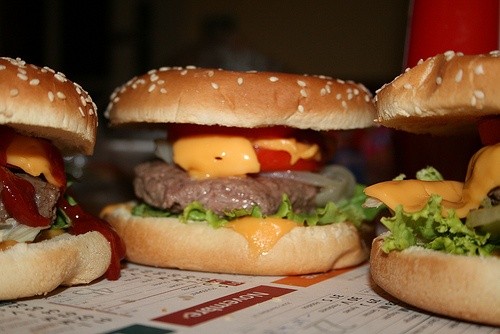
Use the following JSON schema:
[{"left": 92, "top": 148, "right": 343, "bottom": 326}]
[
  {"left": 373, "top": 47, "right": 500, "bottom": 327},
  {"left": 0, "top": 54, "right": 128, "bottom": 301},
  {"left": 99, "top": 66, "right": 377, "bottom": 277}
]
